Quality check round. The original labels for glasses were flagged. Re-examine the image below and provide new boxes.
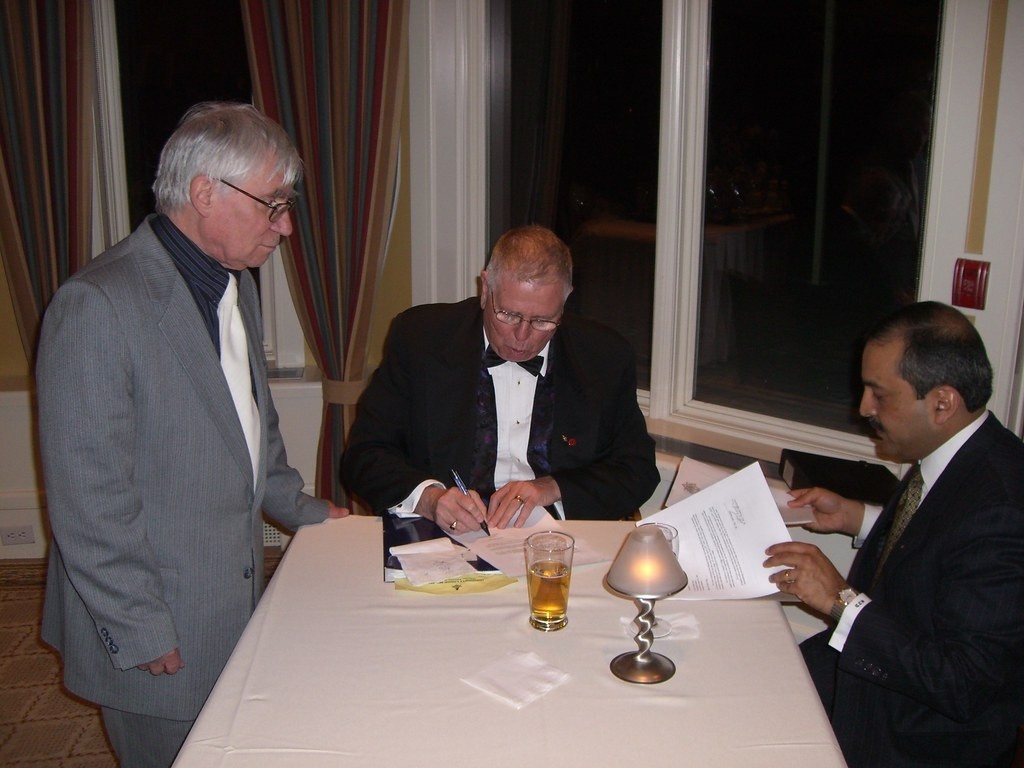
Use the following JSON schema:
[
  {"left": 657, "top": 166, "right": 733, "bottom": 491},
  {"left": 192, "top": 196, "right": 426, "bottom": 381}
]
[
  {"left": 490, "top": 291, "right": 564, "bottom": 331},
  {"left": 214, "top": 176, "right": 299, "bottom": 223}
]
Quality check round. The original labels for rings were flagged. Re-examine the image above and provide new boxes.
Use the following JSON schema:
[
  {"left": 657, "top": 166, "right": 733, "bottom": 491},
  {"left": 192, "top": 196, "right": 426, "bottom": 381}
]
[
  {"left": 786, "top": 569, "right": 791, "bottom": 576},
  {"left": 515, "top": 495, "right": 524, "bottom": 504},
  {"left": 449, "top": 521, "right": 457, "bottom": 530}
]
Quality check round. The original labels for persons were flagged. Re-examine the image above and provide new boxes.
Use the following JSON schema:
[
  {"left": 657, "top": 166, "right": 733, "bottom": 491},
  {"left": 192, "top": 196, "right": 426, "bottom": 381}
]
[
  {"left": 340, "top": 224, "right": 660, "bottom": 537},
  {"left": 34, "top": 103, "right": 354, "bottom": 768},
  {"left": 763, "top": 299, "right": 1024, "bottom": 768}
]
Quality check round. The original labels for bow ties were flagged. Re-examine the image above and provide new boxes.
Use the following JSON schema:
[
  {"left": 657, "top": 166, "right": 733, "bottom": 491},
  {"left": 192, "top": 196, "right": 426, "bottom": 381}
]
[{"left": 484, "top": 343, "right": 545, "bottom": 377}]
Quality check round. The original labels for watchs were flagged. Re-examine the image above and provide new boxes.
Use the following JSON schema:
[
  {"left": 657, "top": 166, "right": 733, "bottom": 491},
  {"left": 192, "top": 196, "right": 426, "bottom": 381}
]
[{"left": 830, "top": 585, "right": 859, "bottom": 623}]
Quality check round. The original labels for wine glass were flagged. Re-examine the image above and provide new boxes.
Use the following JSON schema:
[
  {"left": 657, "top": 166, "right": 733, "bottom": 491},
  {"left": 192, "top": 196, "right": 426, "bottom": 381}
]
[{"left": 629, "top": 523, "right": 679, "bottom": 639}]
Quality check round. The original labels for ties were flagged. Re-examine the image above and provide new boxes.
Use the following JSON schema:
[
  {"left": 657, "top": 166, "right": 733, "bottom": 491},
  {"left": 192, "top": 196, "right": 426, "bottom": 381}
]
[
  {"left": 867, "top": 465, "right": 924, "bottom": 598},
  {"left": 216, "top": 272, "right": 261, "bottom": 493}
]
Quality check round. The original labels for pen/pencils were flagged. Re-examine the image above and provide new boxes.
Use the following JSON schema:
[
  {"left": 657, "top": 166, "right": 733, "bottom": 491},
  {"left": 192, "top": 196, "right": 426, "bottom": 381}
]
[{"left": 448, "top": 468, "right": 492, "bottom": 537}]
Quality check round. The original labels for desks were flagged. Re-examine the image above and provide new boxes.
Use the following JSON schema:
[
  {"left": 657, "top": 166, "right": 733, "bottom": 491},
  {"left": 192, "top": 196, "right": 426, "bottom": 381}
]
[{"left": 171, "top": 514, "right": 849, "bottom": 768}]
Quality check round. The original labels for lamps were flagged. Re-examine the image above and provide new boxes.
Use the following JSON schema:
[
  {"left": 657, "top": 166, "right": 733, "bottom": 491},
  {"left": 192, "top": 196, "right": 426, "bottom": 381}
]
[{"left": 604, "top": 524, "right": 688, "bottom": 684}]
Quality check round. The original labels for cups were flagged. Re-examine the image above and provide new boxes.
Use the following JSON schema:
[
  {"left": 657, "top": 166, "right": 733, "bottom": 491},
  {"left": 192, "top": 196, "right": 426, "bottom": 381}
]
[{"left": 526, "top": 531, "right": 575, "bottom": 631}]
